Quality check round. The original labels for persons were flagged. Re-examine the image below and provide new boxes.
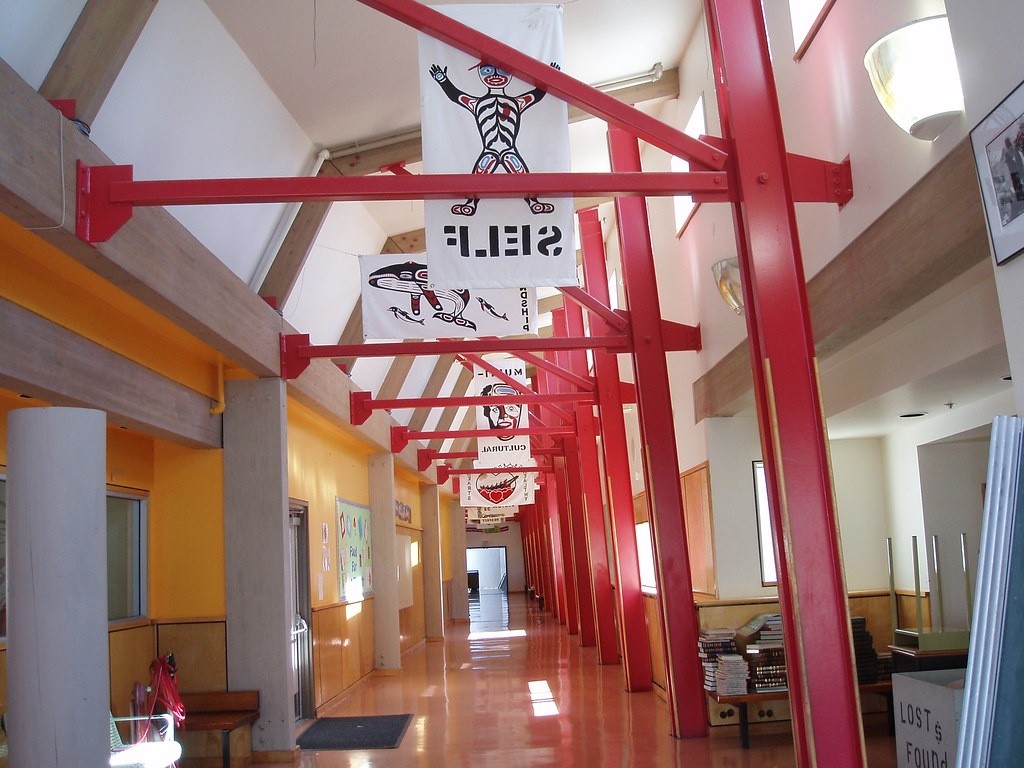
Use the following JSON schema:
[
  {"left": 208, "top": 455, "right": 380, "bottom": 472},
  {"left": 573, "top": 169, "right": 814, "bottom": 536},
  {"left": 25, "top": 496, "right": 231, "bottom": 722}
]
[{"left": 1003, "top": 136, "right": 1024, "bottom": 200}]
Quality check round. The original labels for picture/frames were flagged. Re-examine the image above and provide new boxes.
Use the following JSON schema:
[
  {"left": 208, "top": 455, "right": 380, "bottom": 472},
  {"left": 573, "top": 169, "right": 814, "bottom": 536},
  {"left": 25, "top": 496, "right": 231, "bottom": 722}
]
[{"left": 968, "top": 80, "right": 1024, "bottom": 266}]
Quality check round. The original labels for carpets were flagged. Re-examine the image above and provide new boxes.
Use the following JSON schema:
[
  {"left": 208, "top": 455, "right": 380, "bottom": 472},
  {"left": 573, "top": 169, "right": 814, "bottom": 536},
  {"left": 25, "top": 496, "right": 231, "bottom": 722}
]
[{"left": 297, "top": 713, "right": 415, "bottom": 750}]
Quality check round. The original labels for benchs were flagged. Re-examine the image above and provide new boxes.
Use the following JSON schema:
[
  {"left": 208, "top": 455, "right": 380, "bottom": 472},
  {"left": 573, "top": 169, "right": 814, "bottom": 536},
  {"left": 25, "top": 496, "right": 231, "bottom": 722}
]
[{"left": 155, "top": 690, "right": 261, "bottom": 768}]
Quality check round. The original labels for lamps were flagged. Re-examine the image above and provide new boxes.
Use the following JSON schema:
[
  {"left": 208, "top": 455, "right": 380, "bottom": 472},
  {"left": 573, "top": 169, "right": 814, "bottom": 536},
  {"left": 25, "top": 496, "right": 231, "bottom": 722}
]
[
  {"left": 711, "top": 256, "right": 745, "bottom": 314},
  {"left": 868, "top": 14, "right": 964, "bottom": 141}
]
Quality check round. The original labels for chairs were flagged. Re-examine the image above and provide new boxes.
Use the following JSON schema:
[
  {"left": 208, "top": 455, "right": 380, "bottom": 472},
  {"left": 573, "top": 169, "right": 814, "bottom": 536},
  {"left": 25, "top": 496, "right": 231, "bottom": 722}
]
[{"left": 109, "top": 710, "right": 183, "bottom": 768}]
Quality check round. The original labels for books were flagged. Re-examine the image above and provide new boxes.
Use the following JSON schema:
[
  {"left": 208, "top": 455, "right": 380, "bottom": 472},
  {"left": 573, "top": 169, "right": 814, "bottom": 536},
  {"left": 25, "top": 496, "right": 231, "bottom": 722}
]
[
  {"left": 851, "top": 616, "right": 892, "bottom": 685},
  {"left": 697, "top": 612, "right": 788, "bottom": 696}
]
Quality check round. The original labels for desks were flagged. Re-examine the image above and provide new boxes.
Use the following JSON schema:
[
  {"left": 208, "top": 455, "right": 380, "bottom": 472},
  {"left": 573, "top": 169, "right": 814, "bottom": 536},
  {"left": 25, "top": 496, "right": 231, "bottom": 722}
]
[
  {"left": 712, "top": 682, "right": 892, "bottom": 750},
  {"left": 886, "top": 534, "right": 973, "bottom": 654},
  {"left": 888, "top": 648, "right": 969, "bottom": 672}
]
